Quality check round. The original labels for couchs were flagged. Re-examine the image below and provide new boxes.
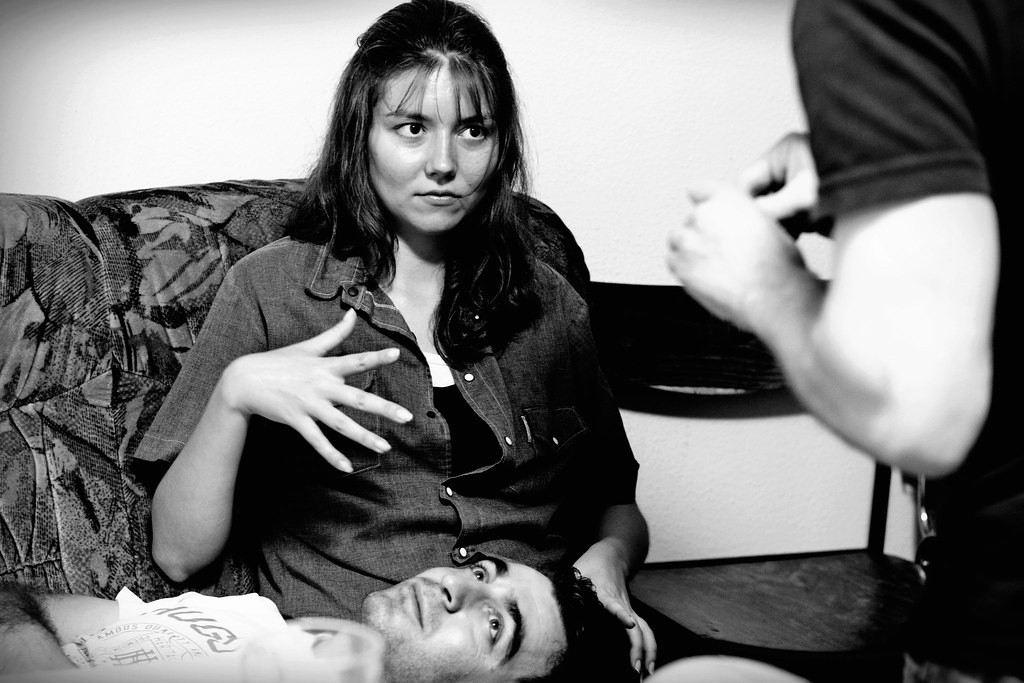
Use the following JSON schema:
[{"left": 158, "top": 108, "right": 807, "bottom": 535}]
[{"left": 4, "top": 180, "right": 599, "bottom": 604}]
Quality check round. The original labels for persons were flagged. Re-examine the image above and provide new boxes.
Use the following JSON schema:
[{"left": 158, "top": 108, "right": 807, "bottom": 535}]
[
  {"left": 0, "top": 550, "right": 652, "bottom": 682},
  {"left": 132, "top": 0, "right": 827, "bottom": 683},
  {"left": 668, "top": 0, "right": 1024, "bottom": 683}
]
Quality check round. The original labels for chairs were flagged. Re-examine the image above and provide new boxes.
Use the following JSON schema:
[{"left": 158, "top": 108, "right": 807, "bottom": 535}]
[{"left": 583, "top": 279, "right": 913, "bottom": 682}]
